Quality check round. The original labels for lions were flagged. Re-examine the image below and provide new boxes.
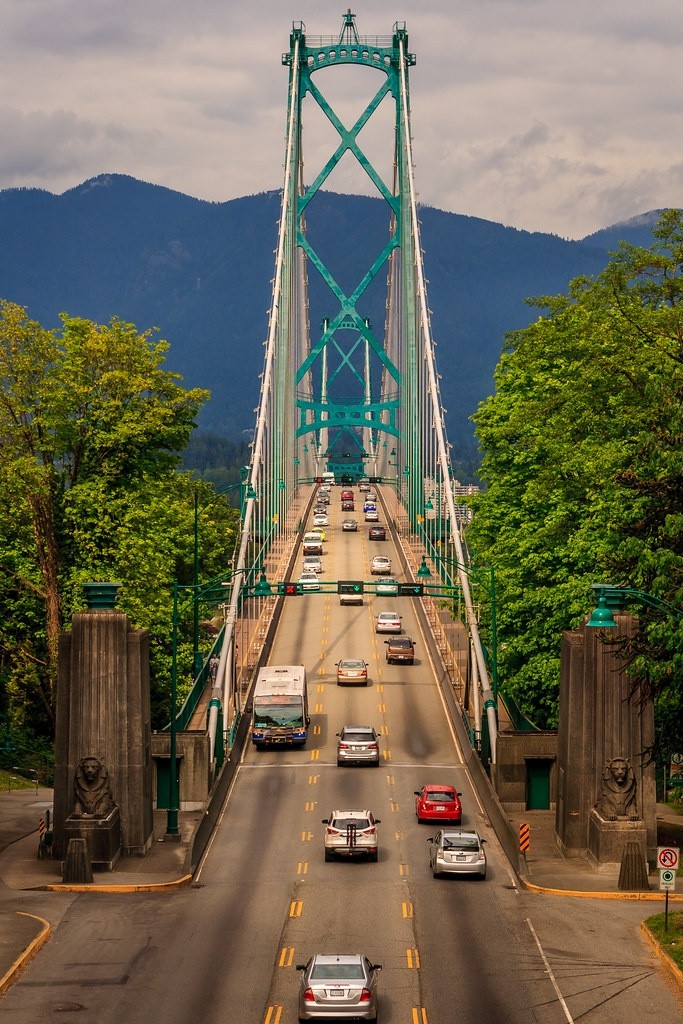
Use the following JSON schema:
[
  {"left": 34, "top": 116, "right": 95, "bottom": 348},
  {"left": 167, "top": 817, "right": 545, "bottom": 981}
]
[
  {"left": 596, "top": 756, "right": 639, "bottom": 821},
  {"left": 72, "top": 755, "right": 115, "bottom": 819}
]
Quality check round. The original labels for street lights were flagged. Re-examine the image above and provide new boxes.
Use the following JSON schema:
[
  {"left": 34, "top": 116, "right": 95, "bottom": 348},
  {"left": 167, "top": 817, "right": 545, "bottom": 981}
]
[
  {"left": 162, "top": 566, "right": 273, "bottom": 842},
  {"left": 193, "top": 480, "right": 256, "bottom": 683},
  {"left": 417, "top": 555, "right": 498, "bottom": 729}
]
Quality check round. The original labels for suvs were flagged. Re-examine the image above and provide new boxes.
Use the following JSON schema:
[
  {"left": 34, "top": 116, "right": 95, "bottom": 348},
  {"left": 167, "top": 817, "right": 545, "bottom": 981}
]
[
  {"left": 321, "top": 808, "right": 381, "bottom": 862},
  {"left": 384, "top": 635, "right": 416, "bottom": 665},
  {"left": 369, "top": 525, "right": 387, "bottom": 541},
  {"left": 335, "top": 725, "right": 381, "bottom": 767}
]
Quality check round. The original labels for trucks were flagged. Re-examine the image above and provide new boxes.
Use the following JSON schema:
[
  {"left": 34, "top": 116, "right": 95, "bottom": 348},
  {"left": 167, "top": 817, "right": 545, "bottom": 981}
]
[{"left": 301, "top": 532, "right": 325, "bottom": 556}]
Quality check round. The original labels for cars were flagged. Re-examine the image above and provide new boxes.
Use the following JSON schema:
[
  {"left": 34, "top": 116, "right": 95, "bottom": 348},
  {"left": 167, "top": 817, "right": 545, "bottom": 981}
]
[
  {"left": 342, "top": 482, "right": 352, "bottom": 487},
  {"left": 375, "top": 577, "right": 398, "bottom": 597},
  {"left": 301, "top": 556, "right": 322, "bottom": 573},
  {"left": 339, "top": 593, "right": 363, "bottom": 606},
  {"left": 413, "top": 785, "right": 462, "bottom": 826},
  {"left": 426, "top": 829, "right": 487, "bottom": 881},
  {"left": 340, "top": 490, "right": 355, "bottom": 511},
  {"left": 296, "top": 952, "right": 382, "bottom": 1024},
  {"left": 312, "top": 483, "right": 331, "bottom": 526},
  {"left": 311, "top": 527, "right": 326, "bottom": 542},
  {"left": 297, "top": 572, "right": 321, "bottom": 590},
  {"left": 342, "top": 519, "right": 358, "bottom": 531},
  {"left": 374, "top": 612, "right": 403, "bottom": 634},
  {"left": 357, "top": 480, "right": 379, "bottom": 522}
]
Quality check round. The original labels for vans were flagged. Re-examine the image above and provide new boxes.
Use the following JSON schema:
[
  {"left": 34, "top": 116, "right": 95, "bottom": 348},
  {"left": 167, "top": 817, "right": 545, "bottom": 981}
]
[
  {"left": 369, "top": 556, "right": 392, "bottom": 575},
  {"left": 335, "top": 659, "right": 369, "bottom": 686}
]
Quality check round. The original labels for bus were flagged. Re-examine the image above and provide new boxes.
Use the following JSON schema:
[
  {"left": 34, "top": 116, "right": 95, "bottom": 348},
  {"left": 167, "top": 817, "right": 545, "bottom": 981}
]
[{"left": 247, "top": 666, "right": 311, "bottom": 749}]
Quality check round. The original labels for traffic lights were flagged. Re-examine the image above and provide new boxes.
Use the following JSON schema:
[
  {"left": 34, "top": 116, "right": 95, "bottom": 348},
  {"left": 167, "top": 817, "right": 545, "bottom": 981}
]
[
  {"left": 284, "top": 583, "right": 296, "bottom": 596},
  {"left": 317, "top": 477, "right": 322, "bottom": 483},
  {"left": 401, "top": 587, "right": 422, "bottom": 594},
  {"left": 324, "top": 453, "right": 331, "bottom": 457},
  {"left": 341, "top": 584, "right": 361, "bottom": 593},
  {"left": 342, "top": 452, "right": 350, "bottom": 457},
  {"left": 361, "top": 454, "right": 369, "bottom": 458},
  {"left": 342, "top": 477, "right": 353, "bottom": 482},
  {"left": 370, "top": 477, "right": 381, "bottom": 483}
]
